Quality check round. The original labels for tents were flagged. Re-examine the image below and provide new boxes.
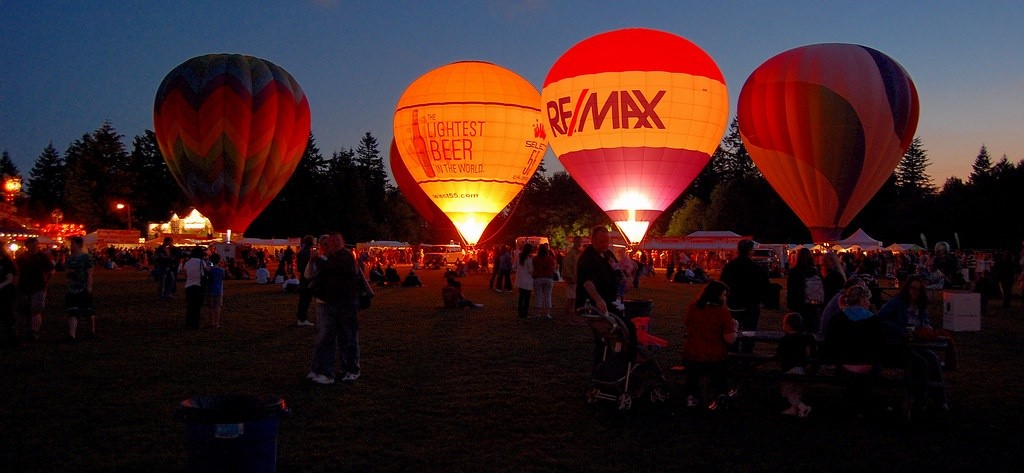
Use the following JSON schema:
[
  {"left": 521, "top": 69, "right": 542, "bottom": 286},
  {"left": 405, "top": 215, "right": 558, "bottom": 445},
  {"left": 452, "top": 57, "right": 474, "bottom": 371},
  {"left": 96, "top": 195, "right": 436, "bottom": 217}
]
[{"left": 636, "top": 227, "right": 926, "bottom": 254}]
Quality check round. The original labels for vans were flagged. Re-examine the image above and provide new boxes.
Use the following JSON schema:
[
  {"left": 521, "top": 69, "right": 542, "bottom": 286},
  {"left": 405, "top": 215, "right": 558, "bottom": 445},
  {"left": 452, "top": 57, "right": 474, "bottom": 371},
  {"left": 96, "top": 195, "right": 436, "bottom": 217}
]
[
  {"left": 515, "top": 236, "right": 550, "bottom": 258},
  {"left": 423, "top": 245, "right": 462, "bottom": 266}
]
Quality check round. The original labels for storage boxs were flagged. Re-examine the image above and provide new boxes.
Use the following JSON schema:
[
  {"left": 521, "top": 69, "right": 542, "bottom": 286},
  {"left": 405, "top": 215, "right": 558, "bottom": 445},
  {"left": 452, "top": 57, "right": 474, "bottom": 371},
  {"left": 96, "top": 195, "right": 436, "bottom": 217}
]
[{"left": 943, "top": 292, "right": 981, "bottom": 332}]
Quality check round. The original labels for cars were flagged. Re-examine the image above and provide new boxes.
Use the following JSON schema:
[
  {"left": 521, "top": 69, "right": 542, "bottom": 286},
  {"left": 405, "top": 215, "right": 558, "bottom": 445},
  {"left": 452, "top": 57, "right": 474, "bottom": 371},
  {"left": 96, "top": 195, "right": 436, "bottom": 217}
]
[{"left": 750, "top": 249, "right": 778, "bottom": 268}]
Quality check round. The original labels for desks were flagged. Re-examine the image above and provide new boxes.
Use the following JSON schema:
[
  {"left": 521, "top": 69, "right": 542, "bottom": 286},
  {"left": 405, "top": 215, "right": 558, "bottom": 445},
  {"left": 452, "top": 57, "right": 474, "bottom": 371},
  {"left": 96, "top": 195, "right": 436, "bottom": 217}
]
[{"left": 734, "top": 331, "right": 950, "bottom": 415}]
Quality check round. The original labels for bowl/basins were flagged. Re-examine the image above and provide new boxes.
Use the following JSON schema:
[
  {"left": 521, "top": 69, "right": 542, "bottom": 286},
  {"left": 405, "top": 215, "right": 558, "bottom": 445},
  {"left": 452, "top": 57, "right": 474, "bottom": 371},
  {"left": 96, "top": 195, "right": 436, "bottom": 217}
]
[{"left": 741, "top": 331, "right": 755, "bottom": 336}]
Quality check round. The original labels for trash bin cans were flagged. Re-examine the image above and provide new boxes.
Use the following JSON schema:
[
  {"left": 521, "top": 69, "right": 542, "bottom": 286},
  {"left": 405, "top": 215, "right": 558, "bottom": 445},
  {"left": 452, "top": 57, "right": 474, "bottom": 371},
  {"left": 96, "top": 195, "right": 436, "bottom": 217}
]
[
  {"left": 623, "top": 299, "right": 653, "bottom": 351},
  {"left": 180, "top": 392, "right": 286, "bottom": 473},
  {"left": 764, "top": 283, "right": 783, "bottom": 309}
]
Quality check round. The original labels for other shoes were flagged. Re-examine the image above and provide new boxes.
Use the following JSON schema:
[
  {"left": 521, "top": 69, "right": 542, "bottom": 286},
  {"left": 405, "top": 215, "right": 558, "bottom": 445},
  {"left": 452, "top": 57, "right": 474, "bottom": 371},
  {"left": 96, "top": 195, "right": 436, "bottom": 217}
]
[
  {"left": 545, "top": 313, "right": 551, "bottom": 319},
  {"left": 495, "top": 287, "right": 502, "bottom": 293},
  {"left": 308, "top": 371, "right": 335, "bottom": 385},
  {"left": 205, "top": 323, "right": 219, "bottom": 328},
  {"left": 87, "top": 332, "right": 96, "bottom": 340},
  {"left": 686, "top": 397, "right": 695, "bottom": 406},
  {"left": 781, "top": 405, "right": 811, "bottom": 418},
  {"left": 536, "top": 315, "right": 540, "bottom": 320},
  {"left": 343, "top": 370, "right": 359, "bottom": 380},
  {"left": 297, "top": 318, "right": 315, "bottom": 326},
  {"left": 717, "top": 395, "right": 727, "bottom": 411}
]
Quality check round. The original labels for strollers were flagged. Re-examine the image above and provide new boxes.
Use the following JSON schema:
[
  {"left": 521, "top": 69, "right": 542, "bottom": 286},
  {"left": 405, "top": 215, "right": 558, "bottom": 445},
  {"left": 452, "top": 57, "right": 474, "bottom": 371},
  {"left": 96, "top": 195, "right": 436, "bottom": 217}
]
[{"left": 576, "top": 307, "right": 670, "bottom": 412}]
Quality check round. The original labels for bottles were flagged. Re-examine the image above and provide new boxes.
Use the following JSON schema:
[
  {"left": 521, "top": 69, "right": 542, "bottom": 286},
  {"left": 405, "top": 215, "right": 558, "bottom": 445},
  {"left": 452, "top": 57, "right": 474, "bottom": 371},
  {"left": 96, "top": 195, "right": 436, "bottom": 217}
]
[{"left": 584, "top": 299, "right": 592, "bottom": 316}]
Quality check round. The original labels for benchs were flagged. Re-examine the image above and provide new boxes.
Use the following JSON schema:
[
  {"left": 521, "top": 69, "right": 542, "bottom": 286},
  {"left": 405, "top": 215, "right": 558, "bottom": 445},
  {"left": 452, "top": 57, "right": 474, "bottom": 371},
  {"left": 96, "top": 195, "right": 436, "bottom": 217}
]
[{"left": 722, "top": 352, "right": 917, "bottom": 421}]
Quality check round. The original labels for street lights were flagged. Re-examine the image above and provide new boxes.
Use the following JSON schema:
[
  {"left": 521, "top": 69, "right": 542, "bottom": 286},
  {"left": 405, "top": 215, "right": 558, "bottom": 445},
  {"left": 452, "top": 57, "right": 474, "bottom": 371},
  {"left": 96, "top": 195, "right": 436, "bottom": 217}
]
[{"left": 117, "top": 204, "right": 131, "bottom": 230}]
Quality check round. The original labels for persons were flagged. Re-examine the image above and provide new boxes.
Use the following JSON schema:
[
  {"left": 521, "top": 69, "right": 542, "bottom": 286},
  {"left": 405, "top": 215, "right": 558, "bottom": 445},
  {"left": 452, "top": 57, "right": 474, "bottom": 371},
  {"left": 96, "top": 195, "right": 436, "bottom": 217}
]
[{"left": 0, "top": 232, "right": 1024, "bottom": 473}]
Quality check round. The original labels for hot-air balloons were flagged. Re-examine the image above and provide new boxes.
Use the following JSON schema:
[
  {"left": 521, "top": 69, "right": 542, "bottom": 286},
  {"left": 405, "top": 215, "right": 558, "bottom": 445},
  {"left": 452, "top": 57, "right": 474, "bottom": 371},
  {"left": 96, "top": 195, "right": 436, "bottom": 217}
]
[
  {"left": 390, "top": 60, "right": 549, "bottom": 261},
  {"left": 737, "top": 43, "right": 918, "bottom": 264},
  {"left": 154, "top": 53, "right": 311, "bottom": 259},
  {"left": 541, "top": 28, "right": 728, "bottom": 262}
]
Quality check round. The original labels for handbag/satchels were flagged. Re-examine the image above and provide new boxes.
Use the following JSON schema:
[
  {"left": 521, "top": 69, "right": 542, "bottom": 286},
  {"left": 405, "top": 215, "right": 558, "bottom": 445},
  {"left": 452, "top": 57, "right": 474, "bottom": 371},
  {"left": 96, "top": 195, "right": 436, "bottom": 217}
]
[
  {"left": 198, "top": 261, "right": 210, "bottom": 284},
  {"left": 553, "top": 273, "right": 559, "bottom": 280}
]
